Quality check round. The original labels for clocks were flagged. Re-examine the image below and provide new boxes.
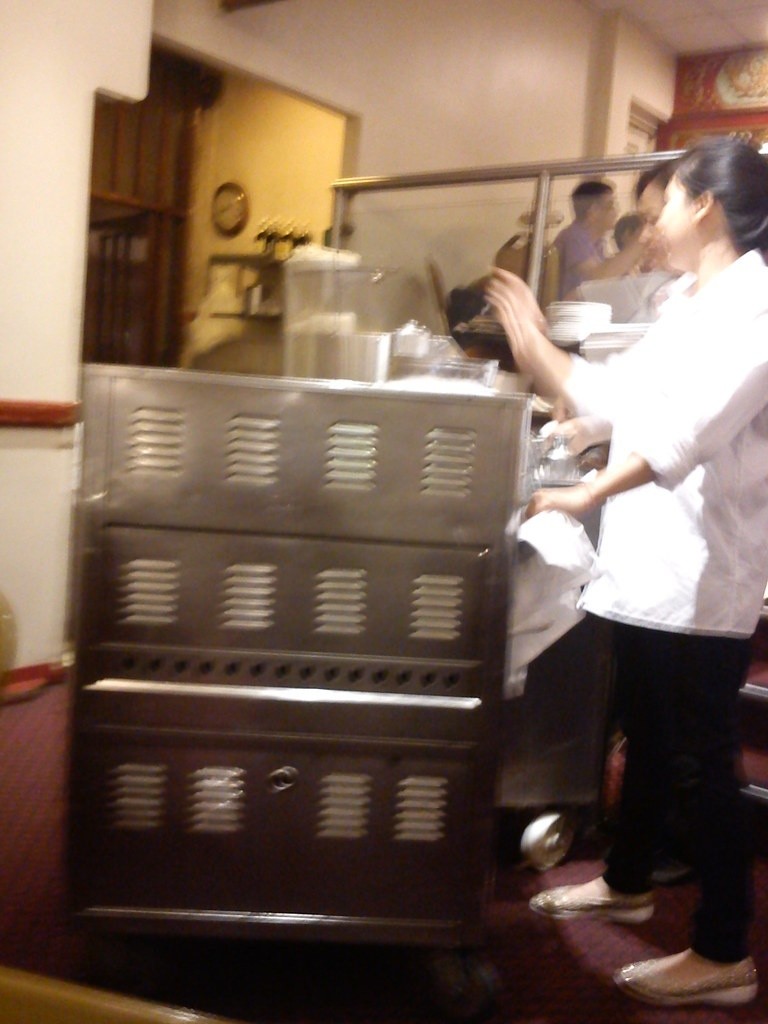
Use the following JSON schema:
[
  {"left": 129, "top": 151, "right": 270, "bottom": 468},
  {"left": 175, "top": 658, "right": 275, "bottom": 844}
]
[{"left": 211, "top": 183, "right": 250, "bottom": 238}]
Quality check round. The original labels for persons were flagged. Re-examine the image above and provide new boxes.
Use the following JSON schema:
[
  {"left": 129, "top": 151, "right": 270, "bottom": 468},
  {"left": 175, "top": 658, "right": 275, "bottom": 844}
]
[
  {"left": 484, "top": 131, "right": 768, "bottom": 1005},
  {"left": 549, "top": 179, "right": 652, "bottom": 302}
]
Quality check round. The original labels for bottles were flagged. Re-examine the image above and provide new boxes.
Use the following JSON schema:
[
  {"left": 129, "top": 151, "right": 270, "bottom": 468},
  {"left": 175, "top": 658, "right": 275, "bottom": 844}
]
[{"left": 537, "top": 438, "right": 578, "bottom": 484}]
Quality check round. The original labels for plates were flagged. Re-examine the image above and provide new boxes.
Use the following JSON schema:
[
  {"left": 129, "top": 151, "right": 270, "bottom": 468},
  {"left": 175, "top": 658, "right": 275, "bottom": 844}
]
[{"left": 546, "top": 302, "right": 655, "bottom": 347}]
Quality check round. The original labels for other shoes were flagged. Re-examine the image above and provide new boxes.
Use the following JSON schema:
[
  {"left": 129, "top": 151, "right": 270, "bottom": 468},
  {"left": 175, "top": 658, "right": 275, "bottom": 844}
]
[
  {"left": 529, "top": 876, "right": 655, "bottom": 925},
  {"left": 613, "top": 949, "right": 759, "bottom": 1006}
]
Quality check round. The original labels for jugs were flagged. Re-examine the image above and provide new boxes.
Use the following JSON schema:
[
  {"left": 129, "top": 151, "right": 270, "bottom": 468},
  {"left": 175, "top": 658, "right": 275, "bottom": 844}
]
[{"left": 284, "top": 244, "right": 362, "bottom": 378}]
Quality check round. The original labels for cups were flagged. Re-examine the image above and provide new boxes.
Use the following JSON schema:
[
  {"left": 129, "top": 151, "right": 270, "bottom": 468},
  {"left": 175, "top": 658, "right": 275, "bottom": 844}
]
[{"left": 346, "top": 333, "right": 390, "bottom": 383}]
